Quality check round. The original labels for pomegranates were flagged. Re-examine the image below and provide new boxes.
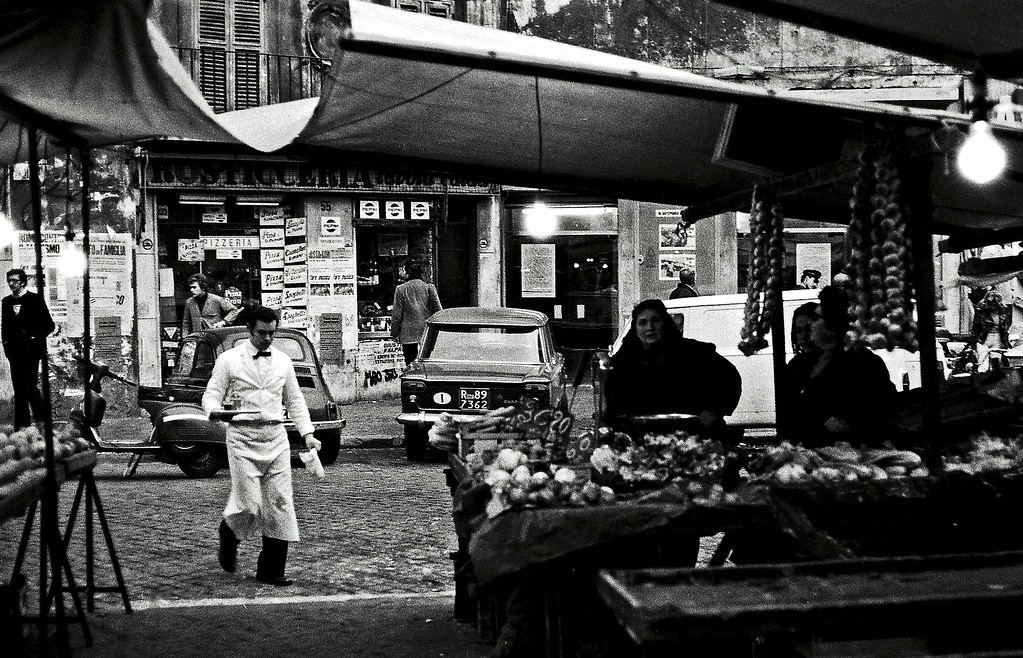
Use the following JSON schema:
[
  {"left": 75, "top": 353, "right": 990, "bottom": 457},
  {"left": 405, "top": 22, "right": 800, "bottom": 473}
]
[{"left": 0, "top": 425, "right": 90, "bottom": 483}]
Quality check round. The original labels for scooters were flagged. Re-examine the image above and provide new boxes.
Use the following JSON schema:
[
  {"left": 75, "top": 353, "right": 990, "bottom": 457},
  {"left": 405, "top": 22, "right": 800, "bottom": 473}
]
[{"left": 62, "top": 352, "right": 230, "bottom": 478}]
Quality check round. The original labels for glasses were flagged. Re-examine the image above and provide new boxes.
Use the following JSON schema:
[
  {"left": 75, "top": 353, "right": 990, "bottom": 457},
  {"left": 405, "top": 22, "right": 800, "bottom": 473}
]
[{"left": 6, "top": 279, "right": 20, "bottom": 283}]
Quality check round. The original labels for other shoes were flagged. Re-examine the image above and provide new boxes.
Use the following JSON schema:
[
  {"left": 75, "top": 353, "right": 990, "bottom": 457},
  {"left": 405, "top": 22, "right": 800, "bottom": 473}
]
[
  {"left": 256, "top": 574, "right": 293, "bottom": 587},
  {"left": 217, "top": 520, "right": 240, "bottom": 573}
]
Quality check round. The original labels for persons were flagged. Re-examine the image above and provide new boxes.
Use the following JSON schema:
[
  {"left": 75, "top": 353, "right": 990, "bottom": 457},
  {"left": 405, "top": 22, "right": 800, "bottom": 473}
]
[
  {"left": 201, "top": 307, "right": 321, "bottom": 586},
  {"left": 604, "top": 299, "right": 743, "bottom": 444},
  {"left": 777, "top": 288, "right": 896, "bottom": 444},
  {"left": 391, "top": 265, "right": 443, "bottom": 366},
  {"left": 182, "top": 273, "right": 240, "bottom": 364},
  {"left": 798, "top": 270, "right": 821, "bottom": 290},
  {"left": 2, "top": 269, "right": 55, "bottom": 432},
  {"left": 670, "top": 269, "right": 702, "bottom": 299}
]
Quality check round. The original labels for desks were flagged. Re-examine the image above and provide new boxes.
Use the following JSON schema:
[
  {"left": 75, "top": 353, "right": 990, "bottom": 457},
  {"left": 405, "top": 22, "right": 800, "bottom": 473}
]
[
  {"left": 0, "top": 447, "right": 136, "bottom": 658},
  {"left": 447, "top": 453, "right": 1023, "bottom": 658}
]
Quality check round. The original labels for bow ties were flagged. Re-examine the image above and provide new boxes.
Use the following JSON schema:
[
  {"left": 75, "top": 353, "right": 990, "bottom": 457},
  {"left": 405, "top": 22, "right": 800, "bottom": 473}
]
[{"left": 252, "top": 351, "right": 271, "bottom": 360}]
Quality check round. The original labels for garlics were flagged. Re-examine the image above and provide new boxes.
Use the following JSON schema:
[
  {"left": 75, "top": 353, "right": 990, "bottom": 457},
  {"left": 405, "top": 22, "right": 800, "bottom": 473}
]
[
  {"left": 841, "top": 147, "right": 917, "bottom": 351},
  {"left": 738, "top": 201, "right": 785, "bottom": 356}
]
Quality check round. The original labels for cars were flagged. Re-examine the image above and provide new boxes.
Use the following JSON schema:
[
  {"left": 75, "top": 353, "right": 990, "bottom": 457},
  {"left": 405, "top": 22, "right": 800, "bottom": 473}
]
[
  {"left": 607, "top": 288, "right": 948, "bottom": 444},
  {"left": 165, "top": 327, "right": 348, "bottom": 466},
  {"left": 394, "top": 306, "right": 569, "bottom": 462}
]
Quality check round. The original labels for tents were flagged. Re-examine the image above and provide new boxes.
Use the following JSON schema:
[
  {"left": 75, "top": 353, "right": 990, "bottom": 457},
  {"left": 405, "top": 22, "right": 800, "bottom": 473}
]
[{"left": 1, "top": 1, "right": 1023, "bottom": 394}]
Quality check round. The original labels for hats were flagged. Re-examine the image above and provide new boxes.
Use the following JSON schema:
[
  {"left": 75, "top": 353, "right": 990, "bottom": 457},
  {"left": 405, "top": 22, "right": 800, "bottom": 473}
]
[{"left": 802, "top": 270, "right": 821, "bottom": 279}]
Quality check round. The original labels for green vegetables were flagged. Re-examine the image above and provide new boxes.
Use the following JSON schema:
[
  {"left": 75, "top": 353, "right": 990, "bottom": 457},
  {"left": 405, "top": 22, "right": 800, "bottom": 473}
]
[{"left": 769, "top": 432, "right": 1023, "bottom": 483}]
